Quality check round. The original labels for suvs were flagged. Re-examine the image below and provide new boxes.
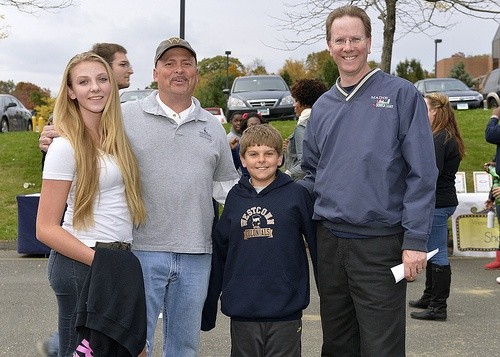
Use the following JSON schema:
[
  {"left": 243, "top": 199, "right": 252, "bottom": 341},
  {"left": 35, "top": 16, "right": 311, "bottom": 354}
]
[{"left": 481, "top": 68, "right": 500, "bottom": 110}]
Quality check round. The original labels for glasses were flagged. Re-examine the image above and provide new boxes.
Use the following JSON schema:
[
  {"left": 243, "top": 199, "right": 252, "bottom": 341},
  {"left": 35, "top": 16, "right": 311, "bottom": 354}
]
[
  {"left": 329, "top": 35, "right": 369, "bottom": 47},
  {"left": 109, "top": 62, "right": 131, "bottom": 68}
]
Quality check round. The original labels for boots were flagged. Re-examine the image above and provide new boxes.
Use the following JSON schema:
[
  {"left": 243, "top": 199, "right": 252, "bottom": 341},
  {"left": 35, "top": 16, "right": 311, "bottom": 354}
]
[
  {"left": 408, "top": 260, "right": 432, "bottom": 309},
  {"left": 410, "top": 262, "right": 450, "bottom": 320},
  {"left": 484, "top": 250, "right": 500, "bottom": 269}
]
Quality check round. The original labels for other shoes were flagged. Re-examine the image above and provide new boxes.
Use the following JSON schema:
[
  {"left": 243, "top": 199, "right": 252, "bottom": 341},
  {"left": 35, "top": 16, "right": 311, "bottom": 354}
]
[
  {"left": 37, "top": 339, "right": 52, "bottom": 357},
  {"left": 496, "top": 277, "right": 500, "bottom": 283}
]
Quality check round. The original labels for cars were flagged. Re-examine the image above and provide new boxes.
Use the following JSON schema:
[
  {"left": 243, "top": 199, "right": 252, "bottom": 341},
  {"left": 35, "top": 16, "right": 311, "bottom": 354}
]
[
  {"left": 119, "top": 88, "right": 154, "bottom": 104},
  {"left": 222, "top": 75, "right": 300, "bottom": 124},
  {"left": 0, "top": 94, "right": 33, "bottom": 134},
  {"left": 204, "top": 107, "right": 228, "bottom": 125},
  {"left": 413, "top": 77, "right": 483, "bottom": 111}
]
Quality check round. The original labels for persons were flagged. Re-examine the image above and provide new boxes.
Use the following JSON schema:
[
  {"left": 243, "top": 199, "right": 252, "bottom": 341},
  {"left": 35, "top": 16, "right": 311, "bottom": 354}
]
[
  {"left": 484, "top": 107, "right": 500, "bottom": 284},
  {"left": 38, "top": 37, "right": 239, "bottom": 357},
  {"left": 38, "top": 42, "right": 134, "bottom": 357},
  {"left": 200, "top": 125, "right": 318, "bottom": 357},
  {"left": 36, "top": 53, "right": 146, "bottom": 357},
  {"left": 409, "top": 94, "right": 465, "bottom": 321},
  {"left": 296, "top": 5, "right": 439, "bottom": 357},
  {"left": 284, "top": 78, "right": 327, "bottom": 183},
  {"left": 227, "top": 112, "right": 269, "bottom": 170}
]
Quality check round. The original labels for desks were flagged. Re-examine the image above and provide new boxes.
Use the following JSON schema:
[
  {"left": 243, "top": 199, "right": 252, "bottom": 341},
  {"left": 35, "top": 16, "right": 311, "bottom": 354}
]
[
  {"left": 16, "top": 193, "right": 68, "bottom": 258},
  {"left": 448, "top": 192, "right": 500, "bottom": 258}
]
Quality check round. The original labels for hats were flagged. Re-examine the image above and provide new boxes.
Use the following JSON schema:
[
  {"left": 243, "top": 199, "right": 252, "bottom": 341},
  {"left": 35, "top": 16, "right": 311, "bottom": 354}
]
[{"left": 155, "top": 37, "right": 197, "bottom": 68}]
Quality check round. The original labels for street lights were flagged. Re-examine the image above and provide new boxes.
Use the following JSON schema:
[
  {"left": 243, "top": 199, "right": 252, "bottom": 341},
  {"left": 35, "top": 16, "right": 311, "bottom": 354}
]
[
  {"left": 224, "top": 50, "right": 231, "bottom": 99},
  {"left": 435, "top": 39, "right": 442, "bottom": 79}
]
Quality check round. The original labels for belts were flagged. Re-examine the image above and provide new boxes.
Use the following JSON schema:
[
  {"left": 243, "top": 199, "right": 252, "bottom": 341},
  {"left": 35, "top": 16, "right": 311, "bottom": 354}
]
[{"left": 92, "top": 242, "right": 131, "bottom": 250}]
